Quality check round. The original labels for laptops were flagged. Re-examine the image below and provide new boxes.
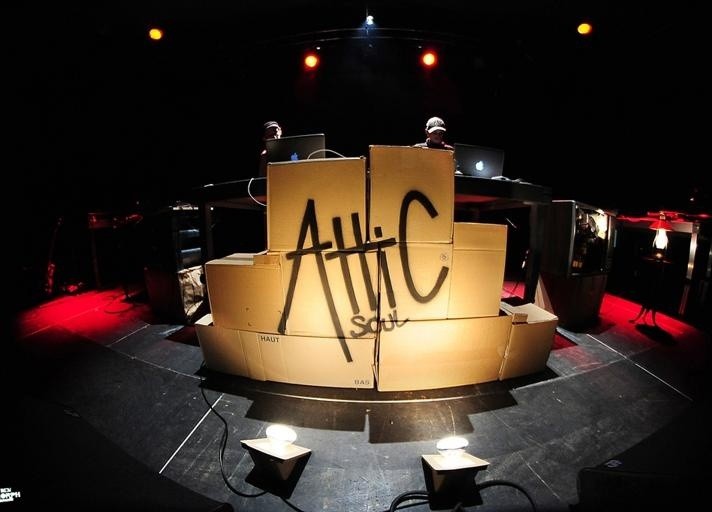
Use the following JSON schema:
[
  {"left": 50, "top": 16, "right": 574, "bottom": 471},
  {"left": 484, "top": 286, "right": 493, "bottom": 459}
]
[
  {"left": 454, "top": 144, "right": 505, "bottom": 179},
  {"left": 265, "top": 134, "right": 326, "bottom": 164}
]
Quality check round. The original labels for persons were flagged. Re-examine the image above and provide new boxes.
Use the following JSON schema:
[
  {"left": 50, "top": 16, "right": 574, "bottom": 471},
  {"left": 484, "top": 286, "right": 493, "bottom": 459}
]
[
  {"left": 259, "top": 121, "right": 298, "bottom": 178},
  {"left": 413, "top": 116, "right": 454, "bottom": 151}
]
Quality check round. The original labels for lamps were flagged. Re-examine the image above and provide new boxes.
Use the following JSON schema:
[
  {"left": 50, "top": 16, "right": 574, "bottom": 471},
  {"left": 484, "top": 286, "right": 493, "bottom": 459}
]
[
  {"left": 241, "top": 424, "right": 311, "bottom": 486},
  {"left": 422, "top": 437, "right": 491, "bottom": 493}
]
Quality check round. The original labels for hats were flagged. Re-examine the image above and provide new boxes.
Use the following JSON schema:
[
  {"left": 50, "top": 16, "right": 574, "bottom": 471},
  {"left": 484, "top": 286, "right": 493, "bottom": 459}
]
[
  {"left": 263, "top": 121, "right": 278, "bottom": 130},
  {"left": 426, "top": 117, "right": 446, "bottom": 133}
]
